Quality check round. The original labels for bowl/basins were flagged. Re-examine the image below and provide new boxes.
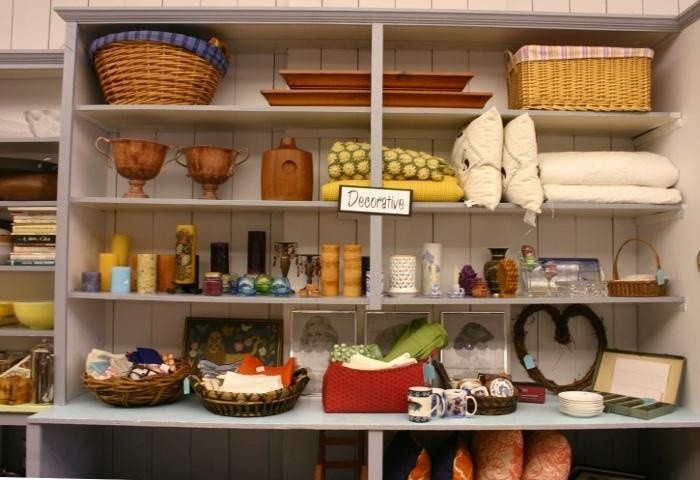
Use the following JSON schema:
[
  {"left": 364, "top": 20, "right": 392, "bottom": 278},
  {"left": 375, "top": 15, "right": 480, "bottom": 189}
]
[{"left": 11, "top": 301, "right": 54, "bottom": 329}]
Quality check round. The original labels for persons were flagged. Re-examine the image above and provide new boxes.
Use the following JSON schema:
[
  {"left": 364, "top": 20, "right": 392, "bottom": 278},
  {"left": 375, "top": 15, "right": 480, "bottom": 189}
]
[
  {"left": 297, "top": 315, "right": 339, "bottom": 354},
  {"left": 452, "top": 321, "right": 495, "bottom": 370}
]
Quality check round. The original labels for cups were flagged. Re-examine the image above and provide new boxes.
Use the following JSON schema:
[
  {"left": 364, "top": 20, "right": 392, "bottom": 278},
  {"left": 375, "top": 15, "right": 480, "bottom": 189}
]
[
  {"left": 444, "top": 389, "right": 477, "bottom": 419},
  {"left": 408, "top": 383, "right": 444, "bottom": 422}
]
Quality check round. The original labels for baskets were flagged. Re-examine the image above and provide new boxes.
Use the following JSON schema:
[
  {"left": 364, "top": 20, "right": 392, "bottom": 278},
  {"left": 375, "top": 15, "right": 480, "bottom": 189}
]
[
  {"left": 192, "top": 366, "right": 309, "bottom": 417},
  {"left": 501, "top": 44, "right": 655, "bottom": 112},
  {"left": 608, "top": 238, "right": 669, "bottom": 297},
  {"left": 88, "top": 30, "right": 229, "bottom": 105},
  {"left": 82, "top": 357, "right": 193, "bottom": 407}
]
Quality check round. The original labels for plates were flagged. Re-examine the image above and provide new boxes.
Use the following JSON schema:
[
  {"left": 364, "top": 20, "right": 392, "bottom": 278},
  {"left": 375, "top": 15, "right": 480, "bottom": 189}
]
[{"left": 558, "top": 390, "right": 605, "bottom": 419}]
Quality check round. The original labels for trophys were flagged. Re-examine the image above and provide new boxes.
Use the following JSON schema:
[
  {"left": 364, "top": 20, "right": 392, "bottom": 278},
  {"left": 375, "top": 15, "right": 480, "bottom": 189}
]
[
  {"left": 174, "top": 143, "right": 250, "bottom": 199},
  {"left": 95, "top": 135, "right": 184, "bottom": 200}
]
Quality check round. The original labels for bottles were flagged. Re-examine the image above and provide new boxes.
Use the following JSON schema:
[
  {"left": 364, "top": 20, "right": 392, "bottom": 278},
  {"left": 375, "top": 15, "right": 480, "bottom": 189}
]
[{"left": 28, "top": 338, "right": 54, "bottom": 405}]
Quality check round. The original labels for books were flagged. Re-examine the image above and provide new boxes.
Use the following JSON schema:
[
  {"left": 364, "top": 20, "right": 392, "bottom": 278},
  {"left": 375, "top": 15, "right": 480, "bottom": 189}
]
[{"left": 3, "top": 206, "right": 57, "bottom": 265}]
